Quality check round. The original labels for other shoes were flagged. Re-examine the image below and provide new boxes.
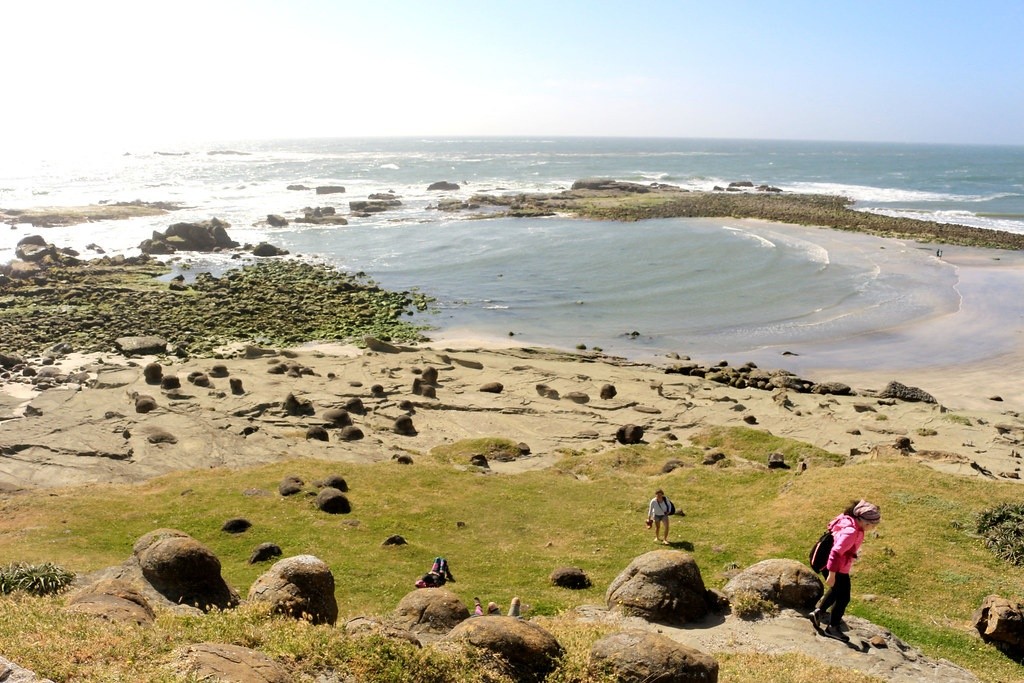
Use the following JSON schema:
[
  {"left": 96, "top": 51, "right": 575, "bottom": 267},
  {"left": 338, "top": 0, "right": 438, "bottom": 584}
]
[
  {"left": 654, "top": 538, "right": 660, "bottom": 541},
  {"left": 663, "top": 540, "right": 669, "bottom": 544},
  {"left": 474, "top": 597, "right": 482, "bottom": 608},
  {"left": 446, "top": 575, "right": 456, "bottom": 582}
]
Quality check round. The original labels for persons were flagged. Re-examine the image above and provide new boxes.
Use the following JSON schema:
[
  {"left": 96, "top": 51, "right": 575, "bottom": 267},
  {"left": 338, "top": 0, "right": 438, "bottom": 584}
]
[
  {"left": 647, "top": 489, "right": 672, "bottom": 545},
  {"left": 807, "top": 498, "right": 881, "bottom": 643},
  {"left": 474, "top": 595, "right": 520, "bottom": 618},
  {"left": 421, "top": 556, "right": 456, "bottom": 586}
]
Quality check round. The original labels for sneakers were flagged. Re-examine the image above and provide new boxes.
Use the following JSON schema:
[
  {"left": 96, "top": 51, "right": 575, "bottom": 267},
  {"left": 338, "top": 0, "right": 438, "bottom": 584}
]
[
  {"left": 825, "top": 624, "right": 849, "bottom": 643},
  {"left": 808, "top": 608, "right": 822, "bottom": 627}
]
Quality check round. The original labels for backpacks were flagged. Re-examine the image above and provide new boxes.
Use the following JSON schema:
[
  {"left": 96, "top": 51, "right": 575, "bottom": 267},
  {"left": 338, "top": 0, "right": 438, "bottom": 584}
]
[{"left": 809, "top": 531, "right": 834, "bottom": 574}]
